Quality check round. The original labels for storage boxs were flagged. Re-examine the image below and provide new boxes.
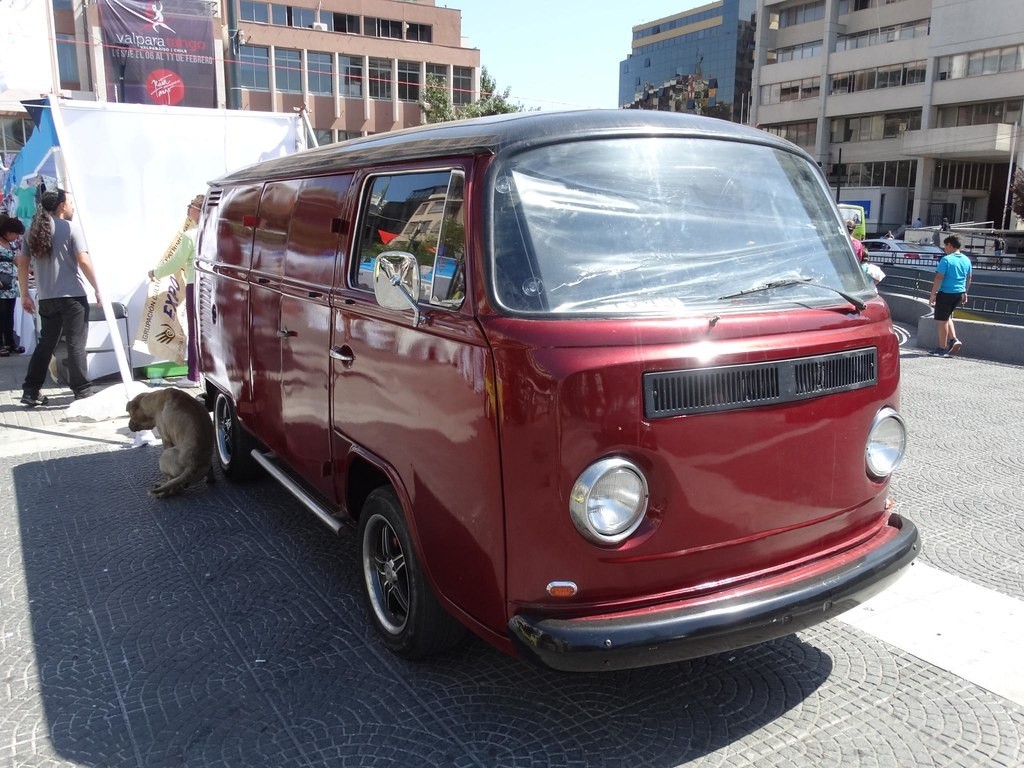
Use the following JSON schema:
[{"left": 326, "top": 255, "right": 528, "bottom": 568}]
[{"left": 141, "top": 360, "right": 189, "bottom": 378}]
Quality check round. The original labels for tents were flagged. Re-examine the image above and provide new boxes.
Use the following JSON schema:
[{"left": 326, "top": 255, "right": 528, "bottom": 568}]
[{"left": 0, "top": 94, "right": 321, "bottom": 447}]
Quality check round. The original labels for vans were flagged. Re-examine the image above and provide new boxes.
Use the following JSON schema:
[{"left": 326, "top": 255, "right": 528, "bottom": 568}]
[{"left": 190, "top": 107, "right": 926, "bottom": 676}]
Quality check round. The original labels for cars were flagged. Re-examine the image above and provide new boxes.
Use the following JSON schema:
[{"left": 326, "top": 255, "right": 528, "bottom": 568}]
[
  {"left": 916, "top": 243, "right": 946, "bottom": 259},
  {"left": 860, "top": 239, "right": 938, "bottom": 267}
]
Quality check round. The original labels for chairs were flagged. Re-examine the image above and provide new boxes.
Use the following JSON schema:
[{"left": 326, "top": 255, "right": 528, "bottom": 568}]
[{"left": 86, "top": 302, "right": 134, "bottom": 379}]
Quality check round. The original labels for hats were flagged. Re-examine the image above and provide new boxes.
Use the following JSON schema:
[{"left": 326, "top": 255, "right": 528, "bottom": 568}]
[
  {"left": 846, "top": 220, "right": 856, "bottom": 228},
  {"left": 943, "top": 218, "right": 948, "bottom": 222},
  {"left": 5, "top": 217, "right": 25, "bottom": 235},
  {"left": 187, "top": 194, "right": 206, "bottom": 211}
]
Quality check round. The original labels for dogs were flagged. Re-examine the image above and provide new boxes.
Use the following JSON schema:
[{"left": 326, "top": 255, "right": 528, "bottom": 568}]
[{"left": 126, "top": 388, "right": 218, "bottom": 499}]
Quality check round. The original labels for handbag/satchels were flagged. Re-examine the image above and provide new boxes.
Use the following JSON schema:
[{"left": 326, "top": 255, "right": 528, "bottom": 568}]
[{"left": 0, "top": 270, "right": 13, "bottom": 289}]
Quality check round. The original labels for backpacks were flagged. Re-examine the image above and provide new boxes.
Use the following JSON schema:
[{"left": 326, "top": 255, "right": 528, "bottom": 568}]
[{"left": 998, "top": 238, "right": 1005, "bottom": 250}]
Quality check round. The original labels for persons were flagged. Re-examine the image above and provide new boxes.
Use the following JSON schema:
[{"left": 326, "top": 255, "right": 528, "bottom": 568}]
[
  {"left": 912, "top": 218, "right": 922, "bottom": 228},
  {"left": 943, "top": 218, "right": 950, "bottom": 231},
  {"left": 844, "top": 219, "right": 886, "bottom": 294},
  {"left": 930, "top": 233, "right": 972, "bottom": 358},
  {"left": 148, "top": 195, "right": 205, "bottom": 388},
  {"left": 880, "top": 230, "right": 894, "bottom": 239},
  {"left": 0, "top": 216, "right": 34, "bottom": 357},
  {"left": 990, "top": 233, "right": 1005, "bottom": 269},
  {"left": 18, "top": 187, "right": 102, "bottom": 406},
  {"left": 853, "top": 214, "right": 861, "bottom": 224}
]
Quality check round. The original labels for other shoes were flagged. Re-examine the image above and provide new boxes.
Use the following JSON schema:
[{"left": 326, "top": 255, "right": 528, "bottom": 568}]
[
  {"left": 72, "top": 382, "right": 103, "bottom": 399},
  {"left": 0, "top": 348, "right": 10, "bottom": 355},
  {"left": 20, "top": 391, "right": 49, "bottom": 405},
  {"left": 927, "top": 347, "right": 954, "bottom": 357},
  {"left": 7, "top": 346, "right": 25, "bottom": 352},
  {"left": 947, "top": 338, "right": 962, "bottom": 355},
  {"left": 176, "top": 375, "right": 202, "bottom": 388}
]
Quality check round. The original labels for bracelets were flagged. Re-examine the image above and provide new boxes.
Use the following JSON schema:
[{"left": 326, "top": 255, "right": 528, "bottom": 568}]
[
  {"left": 151, "top": 270, "right": 154, "bottom": 277},
  {"left": 30, "top": 271, "right": 34, "bottom": 275}
]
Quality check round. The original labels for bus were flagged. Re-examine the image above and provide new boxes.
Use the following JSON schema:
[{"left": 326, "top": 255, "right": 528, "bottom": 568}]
[{"left": 836, "top": 203, "right": 866, "bottom": 242}]
